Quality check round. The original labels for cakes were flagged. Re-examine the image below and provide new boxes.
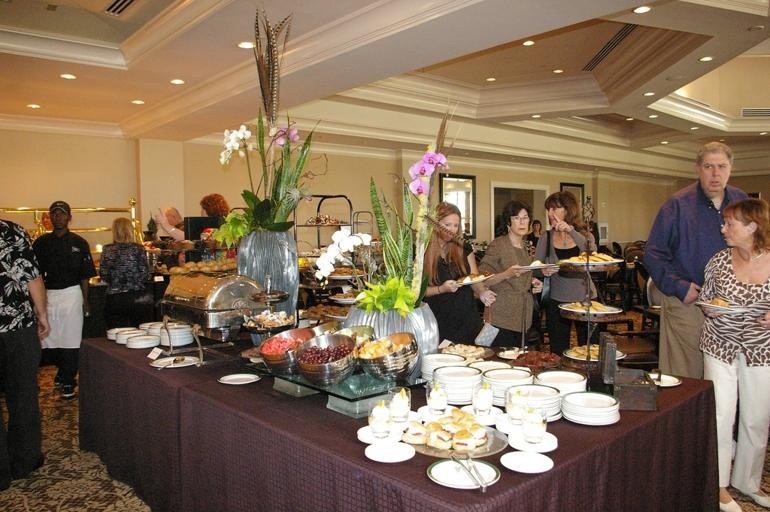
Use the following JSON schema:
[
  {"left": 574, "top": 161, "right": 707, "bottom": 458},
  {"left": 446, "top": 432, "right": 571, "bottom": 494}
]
[{"left": 711, "top": 297, "right": 729, "bottom": 308}]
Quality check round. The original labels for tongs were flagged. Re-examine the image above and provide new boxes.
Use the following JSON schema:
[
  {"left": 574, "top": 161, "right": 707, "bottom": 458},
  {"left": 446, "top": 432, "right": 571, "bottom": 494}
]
[{"left": 450, "top": 452, "right": 488, "bottom": 495}]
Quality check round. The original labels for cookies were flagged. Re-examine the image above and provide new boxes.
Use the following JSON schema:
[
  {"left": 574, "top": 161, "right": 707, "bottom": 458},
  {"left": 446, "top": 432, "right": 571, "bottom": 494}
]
[
  {"left": 569, "top": 252, "right": 615, "bottom": 264},
  {"left": 567, "top": 300, "right": 608, "bottom": 312},
  {"left": 566, "top": 344, "right": 600, "bottom": 361}
]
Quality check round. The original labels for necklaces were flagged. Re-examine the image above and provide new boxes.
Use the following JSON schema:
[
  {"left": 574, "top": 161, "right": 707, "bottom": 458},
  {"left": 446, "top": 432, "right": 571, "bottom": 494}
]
[
  {"left": 737, "top": 248, "right": 764, "bottom": 264},
  {"left": 557, "top": 235, "right": 568, "bottom": 250}
]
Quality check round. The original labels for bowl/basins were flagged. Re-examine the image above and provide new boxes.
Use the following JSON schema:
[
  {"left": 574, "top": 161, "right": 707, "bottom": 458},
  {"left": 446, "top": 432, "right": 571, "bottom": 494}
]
[
  {"left": 292, "top": 334, "right": 356, "bottom": 387},
  {"left": 356, "top": 333, "right": 420, "bottom": 382},
  {"left": 311, "top": 321, "right": 342, "bottom": 338},
  {"left": 261, "top": 328, "right": 316, "bottom": 376},
  {"left": 334, "top": 325, "right": 375, "bottom": 350}
]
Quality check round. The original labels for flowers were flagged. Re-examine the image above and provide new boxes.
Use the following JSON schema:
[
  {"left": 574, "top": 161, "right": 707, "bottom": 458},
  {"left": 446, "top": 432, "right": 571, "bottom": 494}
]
[
  {"left": 211, "top": 8, "right": 327, "bottom": 247},
  {"left": 313, "top": 97, "right": 491, "bottom": 321}
]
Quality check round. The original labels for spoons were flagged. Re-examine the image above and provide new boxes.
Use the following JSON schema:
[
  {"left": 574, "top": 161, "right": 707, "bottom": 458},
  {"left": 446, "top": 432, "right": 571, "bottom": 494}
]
[{"left": 157, "top": 356, "right": 185, "bottom": 371}]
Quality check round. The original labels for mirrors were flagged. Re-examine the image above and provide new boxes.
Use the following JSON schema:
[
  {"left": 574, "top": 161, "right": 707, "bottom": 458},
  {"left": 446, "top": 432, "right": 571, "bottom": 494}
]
[
  {"left": 438, "top": 172, "right": 477, "bottom": 238},
  {"left": 560, "top": 182, "right": 585, "bottom": 231}
]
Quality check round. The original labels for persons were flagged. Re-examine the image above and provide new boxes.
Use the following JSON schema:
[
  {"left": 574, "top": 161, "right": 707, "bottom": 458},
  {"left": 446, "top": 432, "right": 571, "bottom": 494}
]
[
  {"left": 700, "top": 199, "right": 770, "bottom": 512},
  {"left": 99, "top": 218, "right": 151, "bottom": 294},
  {"left": 165, "top": 206, "right": 186, "bottom": 232},
  {"left": 155, "top": 194, "right": 230, "bottom": 254},
  {"left": 0, "top": 218, "right": 49, "bottom": 490},
  {"left": 644, "top": 141, "right": 749, "bottom": 378},
  {"left": 477, "top": 200, "right": 543, "bottom": 347},
  {"left": 528, "top": 220, "right": 542, "bottom": 247},
  {"left": 420, "top": 202, "right": 498, "bottom": 345},
  {"left": 39, "top": 210, "right": 54, "bottom": 234},
  {"left": 33, "top": 200, "right": 97, "bottom": 397},
  {"left": 532, "top": 192, "right": 596, "bottom": 355}
]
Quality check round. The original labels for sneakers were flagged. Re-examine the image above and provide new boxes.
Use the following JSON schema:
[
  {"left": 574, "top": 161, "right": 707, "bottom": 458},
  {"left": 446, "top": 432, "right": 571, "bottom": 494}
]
[
  {"left": 63, "top": 383, "right": 75, "bottom": 399},
  {"left": 53, "top": 376, "right": 64, "bottom": 386}
]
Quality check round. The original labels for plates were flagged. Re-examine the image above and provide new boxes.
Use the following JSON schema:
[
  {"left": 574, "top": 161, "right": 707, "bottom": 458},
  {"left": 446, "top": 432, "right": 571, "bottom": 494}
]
[
  {"left": 321, "top": 312, "right": 347, "bottom": 320},
  {"left": 105, "top": 323, "right": 195, "bottom": 348},
  {"left": 216, "top": 372, "right": 262, "bottom": 386},
  {"left": 647, "top": 373, "right": 683, "bottom": 387},
  {"left": 558, "top": 301, "right": 624, "bottom": 314},
  {"left": 355, "top": 353, "right": 620, "bottom": 489},
  {"left": 497, "top": 350, "right": 530, "bottom": 360},
  {"left": 562, "top": 348, "right": 627, "bottom": 362},
  {"left": 697, "top": 302, "right": 733, "bottom": 312},
  {"left": 455, "top": 273, "right": 495, "bottom": 286},
  {"left": 149, "top": 355, "right": 199, "bottom": 368},
  {"left": 559, "top": 258, "right": 624, "bottom": 266},
  {"left": 523, "top": 264, "right": 556, "bottom": 270},
  {"left": 328, "top": 275, "right": 366, "bottom": 279},
  {"left": 717, "top": 306, "right": 756, "bottom": 315},
  {"left": 328, "top": 295, "right": 356, "bottom": 306}
]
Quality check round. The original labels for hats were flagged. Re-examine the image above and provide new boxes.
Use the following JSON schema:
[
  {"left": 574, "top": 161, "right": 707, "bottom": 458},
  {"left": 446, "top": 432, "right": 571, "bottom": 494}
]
[{"left": 50, "top": 201, "right": 70, "bottom": 214}]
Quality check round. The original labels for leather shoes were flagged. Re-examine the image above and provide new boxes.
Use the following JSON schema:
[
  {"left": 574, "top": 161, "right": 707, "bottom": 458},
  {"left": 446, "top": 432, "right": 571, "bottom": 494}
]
[
  {"left": 750, "top": 491, "right": 770, "bottom": 508},
  {"left": 719, "top": 497, "right": 742, "bottom": 512}
]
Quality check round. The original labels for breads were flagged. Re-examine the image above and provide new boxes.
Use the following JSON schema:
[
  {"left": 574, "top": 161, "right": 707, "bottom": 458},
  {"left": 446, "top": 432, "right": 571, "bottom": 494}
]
[
  {"left": 402, "top": 409, "right": 491, "bottom": 452},
  {"left": 168, "top": 257, "right": 237, "bottom": 274}
]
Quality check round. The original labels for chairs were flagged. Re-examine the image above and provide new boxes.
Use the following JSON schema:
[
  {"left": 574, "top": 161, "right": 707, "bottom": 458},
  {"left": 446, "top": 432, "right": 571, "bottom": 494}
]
[{"left": 574, "top": 320, "right": 660, "bottom": 369}]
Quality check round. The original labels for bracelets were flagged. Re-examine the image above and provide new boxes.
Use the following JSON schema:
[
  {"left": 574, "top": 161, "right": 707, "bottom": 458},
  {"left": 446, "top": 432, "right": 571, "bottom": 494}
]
[
  {"left": 437, "top": 287, "right": 444, "bottom": 298},
  {"left": 166, "top": 228, "right": 176, "bottom": 235}
]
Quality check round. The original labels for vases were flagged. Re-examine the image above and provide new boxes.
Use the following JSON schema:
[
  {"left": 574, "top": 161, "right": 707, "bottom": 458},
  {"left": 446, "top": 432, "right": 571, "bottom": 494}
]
[
  {"left": 343, "top": 303, "right": 439, "bottom": 355},
  {"left": 237, "top": 231, "right": 299, "bottom": 323}
]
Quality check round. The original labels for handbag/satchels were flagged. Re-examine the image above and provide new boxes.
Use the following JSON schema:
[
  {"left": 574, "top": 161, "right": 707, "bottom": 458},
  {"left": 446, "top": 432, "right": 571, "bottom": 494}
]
[
  {"left": 532, "top": 257, "right": 551, "bottom": 301},
  {"left": 474, "top": 323, "right": 499, "bottom": 348}
]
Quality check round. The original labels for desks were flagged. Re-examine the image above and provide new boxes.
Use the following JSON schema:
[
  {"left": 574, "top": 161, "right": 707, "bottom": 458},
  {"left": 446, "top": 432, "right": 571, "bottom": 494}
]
[
  {"left": 180, "top": 340, "right": 721, "bottom": 510},
  {"left": 80, "top": 327, "right": 253, "bottom": 512}
]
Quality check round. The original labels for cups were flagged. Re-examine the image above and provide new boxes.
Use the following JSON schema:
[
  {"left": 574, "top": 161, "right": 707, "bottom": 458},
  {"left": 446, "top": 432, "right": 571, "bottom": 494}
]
[{"left": 367, "top": 381, "right": 548, "bottom": 446}]
